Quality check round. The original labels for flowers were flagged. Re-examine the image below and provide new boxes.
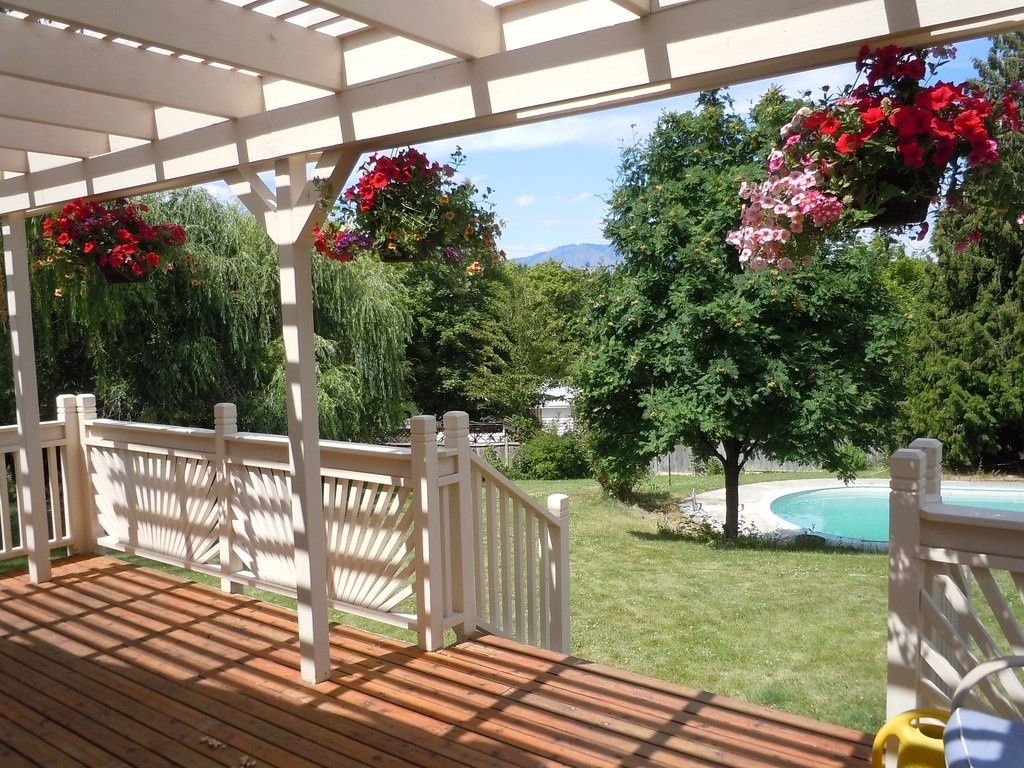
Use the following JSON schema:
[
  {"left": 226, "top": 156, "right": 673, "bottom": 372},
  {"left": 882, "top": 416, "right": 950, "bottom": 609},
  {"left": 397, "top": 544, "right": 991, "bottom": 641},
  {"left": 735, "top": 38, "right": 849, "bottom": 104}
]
[
  {"left": 35, "top": 198, "right": 203, "bottom": 297},
  {"left": 724, "top": 43, "right": 1024, "bottom": 272},
  {"left": 312, "top": 145, "right": 508, "bottom": 276}
]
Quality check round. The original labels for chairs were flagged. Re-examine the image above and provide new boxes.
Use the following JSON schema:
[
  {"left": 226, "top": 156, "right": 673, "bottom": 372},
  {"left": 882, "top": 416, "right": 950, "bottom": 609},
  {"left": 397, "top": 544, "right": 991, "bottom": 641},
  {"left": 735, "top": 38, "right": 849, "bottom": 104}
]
[{"left": 943, "top": 655, "right": 1024, "bottom": 768}]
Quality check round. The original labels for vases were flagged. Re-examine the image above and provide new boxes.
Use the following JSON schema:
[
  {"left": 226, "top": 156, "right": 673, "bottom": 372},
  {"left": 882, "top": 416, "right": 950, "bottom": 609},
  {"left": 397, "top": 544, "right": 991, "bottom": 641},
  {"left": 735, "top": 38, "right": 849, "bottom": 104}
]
[
  {"left": 370, "top": 222, "right": 416, "bottom": 261},
  {"left": 95, "top": 243, "right": 150, "bottom": 283},
  {"left": 840, "top": 166, "right": 946, "bottom": 229}
]
